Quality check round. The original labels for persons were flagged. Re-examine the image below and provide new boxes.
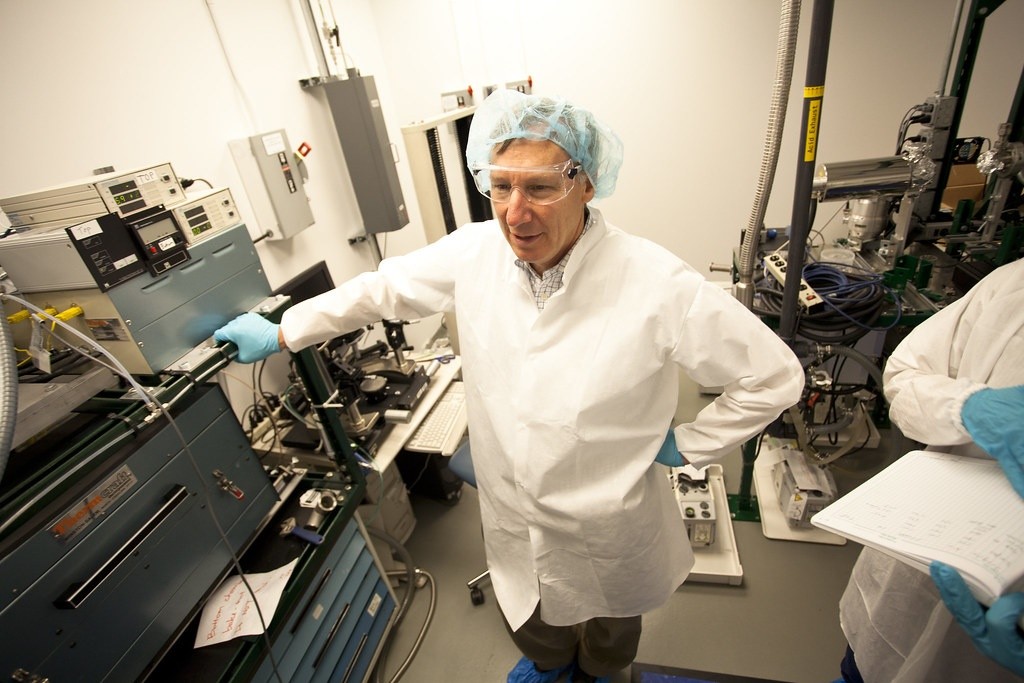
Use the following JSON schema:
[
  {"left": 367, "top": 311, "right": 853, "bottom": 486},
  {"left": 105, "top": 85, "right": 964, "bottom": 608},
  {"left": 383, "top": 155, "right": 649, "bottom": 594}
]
[
  {"left": 213, "top": 85, "right": 807, "bottom": 683},
  {"left": 836, "top": 253, "right": 1024, "bottom": 683}
]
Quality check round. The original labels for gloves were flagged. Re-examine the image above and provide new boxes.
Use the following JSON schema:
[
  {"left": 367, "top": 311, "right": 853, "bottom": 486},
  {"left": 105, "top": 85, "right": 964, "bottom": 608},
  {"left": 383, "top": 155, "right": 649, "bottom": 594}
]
[
  {"left": 930, "top": 561, "right": 1024, "bottom": 677},
  {"left": 961, "top": 385, "right": 1024, "bottom": 502},
  {"left": 654, "top": 429, "right": 684, "bottom": 467},
  {"left": 213, "top": 313, "right": 282, "bottom": 365}
]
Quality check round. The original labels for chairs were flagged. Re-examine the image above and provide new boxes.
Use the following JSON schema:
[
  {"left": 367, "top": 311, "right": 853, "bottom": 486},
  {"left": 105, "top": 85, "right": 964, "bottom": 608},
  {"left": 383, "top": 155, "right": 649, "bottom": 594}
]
[{"left": 449, "top": 442, "right": 492, "bottom": 606}]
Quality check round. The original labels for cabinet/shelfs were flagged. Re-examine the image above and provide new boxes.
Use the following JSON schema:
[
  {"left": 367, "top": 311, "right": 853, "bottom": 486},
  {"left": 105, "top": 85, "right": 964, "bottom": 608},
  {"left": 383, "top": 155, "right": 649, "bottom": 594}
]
[
  {"left": 248, "top": 350, "right": 460, "bottom": 554},
  {"left": 150, "top": 496, "right": 400, "bottom": 683}
]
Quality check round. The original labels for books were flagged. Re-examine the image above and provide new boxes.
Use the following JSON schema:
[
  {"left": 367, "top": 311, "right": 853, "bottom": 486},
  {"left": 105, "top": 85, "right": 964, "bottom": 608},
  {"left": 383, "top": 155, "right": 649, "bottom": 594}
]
[{"left": 807, "top": 449, "right": 1024, "bottom": 632}]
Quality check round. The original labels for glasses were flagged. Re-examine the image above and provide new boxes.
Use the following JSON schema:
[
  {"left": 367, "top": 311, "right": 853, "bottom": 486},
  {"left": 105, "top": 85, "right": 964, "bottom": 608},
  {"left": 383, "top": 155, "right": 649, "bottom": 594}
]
[{"left": 471, "top": 159, "right": 583, "bottom": 205}]
